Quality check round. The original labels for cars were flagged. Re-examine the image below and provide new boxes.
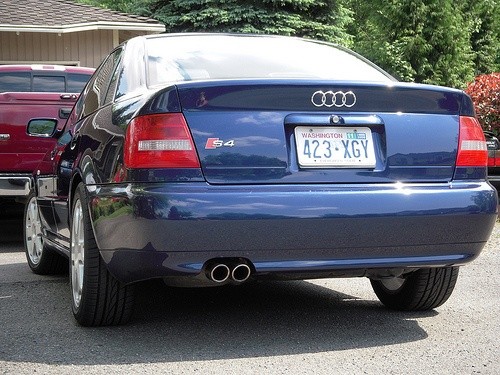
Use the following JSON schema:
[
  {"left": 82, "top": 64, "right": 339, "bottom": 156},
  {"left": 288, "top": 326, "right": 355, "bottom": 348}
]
[
  {"left": 0, "top": 63, "right": 99, "bottom": 212},
  {"left": 21, "top": 32, "right": 498, "bottom": 328}
]
